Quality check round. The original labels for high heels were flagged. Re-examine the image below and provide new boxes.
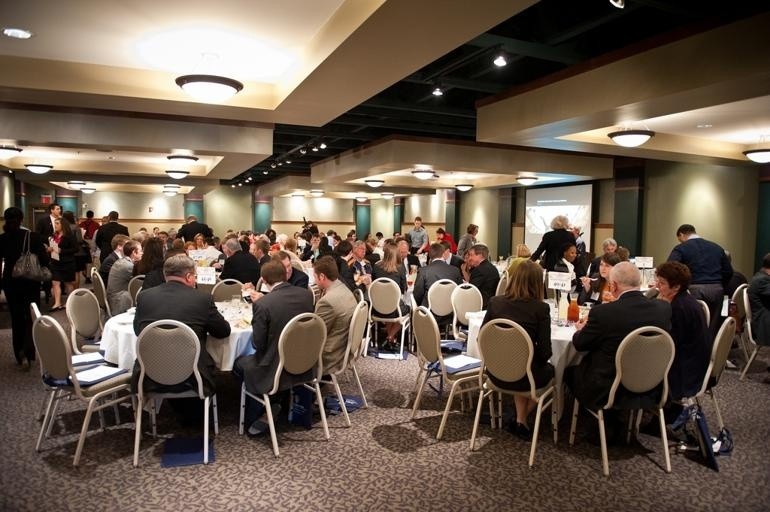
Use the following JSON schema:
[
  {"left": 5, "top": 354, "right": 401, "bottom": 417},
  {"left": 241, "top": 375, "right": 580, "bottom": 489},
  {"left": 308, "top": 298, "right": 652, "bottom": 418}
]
[{"left": 47, "top": 304, "right": 63, "bottom": 312}]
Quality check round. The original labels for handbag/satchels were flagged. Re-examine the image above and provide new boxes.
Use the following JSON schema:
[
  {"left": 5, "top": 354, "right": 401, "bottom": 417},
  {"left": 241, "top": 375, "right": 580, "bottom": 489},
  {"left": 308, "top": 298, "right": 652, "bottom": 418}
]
[
  {"left": 12, "top": 252, "right": 52, "bottom": 282},
  {"left": 424, "top": 338, "right": 465, "bottom": 369}
]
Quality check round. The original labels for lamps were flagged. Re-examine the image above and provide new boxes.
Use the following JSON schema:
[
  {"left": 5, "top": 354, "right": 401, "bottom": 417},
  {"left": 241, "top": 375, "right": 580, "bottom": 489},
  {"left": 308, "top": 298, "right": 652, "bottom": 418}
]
[
  {"left": 175, "top": 75, "right": 243, "bottom": 102},
  {"left": 354, "top": 169, "right": 475, "bottom": 204},
  {"left": 743, "top": 148, "right": 770, "bottom": 164},
  {"left": 162, "top": 170, "right": 190, "bottom": 196},
  {"left": 516, "top": 177, "right": 538, "bottom": 187},
  {"left": 292, "top": 191, "right": 325, "bottom": 200},
  {"left": 432, "top": 87, "right": 444, "bottom": 96},
  {"left": 24, "top": 164, "right": 53, "bottom": 175},
  {"left": 167, "top": 155, "right": 199, "bottom": 165},
  {"left": 0, "top": 147, "right": 23, "bottom": 160},
  {"left": 67, "top": 182, "right": 96, "bottom": 193},
  {"left": 494, "top": 49, "right": 507, "bottom": 67},
  {"left": 607, "top": 130, "right": 655, "bottom": 148},
  {"left": 611, "top": 0, "right": 625, "bottom": 10},
  {"left": 232, "top": 141, "right": 327, "bottom": 187}
]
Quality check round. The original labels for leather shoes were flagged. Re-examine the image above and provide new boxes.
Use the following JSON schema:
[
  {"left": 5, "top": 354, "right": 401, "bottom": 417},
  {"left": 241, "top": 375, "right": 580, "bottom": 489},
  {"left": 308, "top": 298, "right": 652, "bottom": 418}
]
[{"left": 509, "top": 418, "right": 533, "bottom": 443}]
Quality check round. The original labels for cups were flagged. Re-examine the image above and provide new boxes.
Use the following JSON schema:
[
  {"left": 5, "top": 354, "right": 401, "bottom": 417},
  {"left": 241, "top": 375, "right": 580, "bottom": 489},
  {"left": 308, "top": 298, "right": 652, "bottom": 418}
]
[
  {"left": 224, "top": 295, "right": 253, "bottom": 314},
  {"left": 548, "top": 300, "right": 559, "bottom": 325},
  {"left": 499, "top": 255, "right": 504, "bottom": 261}
]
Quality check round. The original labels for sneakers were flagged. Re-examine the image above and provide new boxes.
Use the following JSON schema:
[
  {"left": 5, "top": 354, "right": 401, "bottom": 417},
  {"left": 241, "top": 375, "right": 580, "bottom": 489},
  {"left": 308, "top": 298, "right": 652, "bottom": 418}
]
[
  {"left": 379, "top": 337, "right": 399, "bottom": 354},
  {"left": 15, "top": 359, "right": 35, "bottom": 368},
  {"left": 248, "top": 403, "right": 282, "bottom": 437}
]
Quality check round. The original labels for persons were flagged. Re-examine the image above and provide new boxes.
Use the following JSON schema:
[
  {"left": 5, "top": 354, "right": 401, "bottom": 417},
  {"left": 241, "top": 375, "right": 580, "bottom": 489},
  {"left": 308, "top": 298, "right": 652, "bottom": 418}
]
[
  {"left": 468, "top": 245, "right": 499, "bottom": 310},
  {"left": 555, "top": 224, "right": 770, "bottom": 440},
  {"left": 509, "top": 215, "right": 575, "bottom": 273},
  {"left": 479, "top": 258, "right": 556, "bottom": 440},
  {"left": 2, "top": 203, "right": 479, "bottom": 438}
]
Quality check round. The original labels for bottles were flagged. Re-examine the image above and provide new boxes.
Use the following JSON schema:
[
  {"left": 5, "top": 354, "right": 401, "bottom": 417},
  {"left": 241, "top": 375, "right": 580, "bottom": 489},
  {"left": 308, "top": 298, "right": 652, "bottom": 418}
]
[{"left": 559, "top": 291, "right": 592, "bottom": 328}]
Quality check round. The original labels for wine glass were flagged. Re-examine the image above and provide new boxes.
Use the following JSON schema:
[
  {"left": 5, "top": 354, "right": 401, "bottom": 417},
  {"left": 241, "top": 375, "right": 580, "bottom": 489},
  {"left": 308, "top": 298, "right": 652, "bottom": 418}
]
[{"left": 407, "top": 265, "right": 417, "bottom": 290}]
